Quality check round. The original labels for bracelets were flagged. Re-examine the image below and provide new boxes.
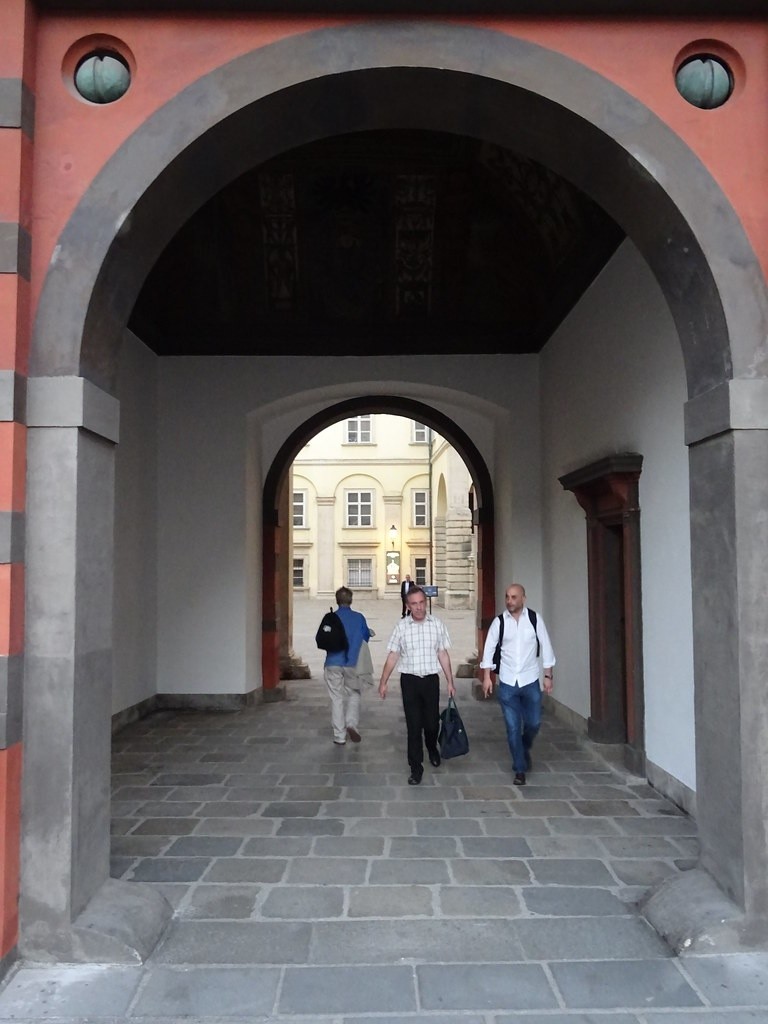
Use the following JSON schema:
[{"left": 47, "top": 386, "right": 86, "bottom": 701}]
[{"left": 545, "top": 674, "right": 553, "bottom": 680}]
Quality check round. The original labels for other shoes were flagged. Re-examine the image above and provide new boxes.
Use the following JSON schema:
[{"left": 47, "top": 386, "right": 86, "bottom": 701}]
[
  {"left": 524, "top": 750, "right": 532, "bottom": 771},
  {"left": 333, "top": 738, "right": 346, "bottom": 745},
  {"left": 346, "top": 724, "right": 361, "bottom": 743},
  {"left": 400, "top": 615, "right": 406, "bottom": 619},
  {"left": 513, "top": 773, "right": 525, "bottom": 785}
]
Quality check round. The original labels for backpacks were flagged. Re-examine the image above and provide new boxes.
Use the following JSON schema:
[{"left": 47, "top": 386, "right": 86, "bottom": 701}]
[{"left": 315, "top": 607, "right": 348, "bottom": 652}]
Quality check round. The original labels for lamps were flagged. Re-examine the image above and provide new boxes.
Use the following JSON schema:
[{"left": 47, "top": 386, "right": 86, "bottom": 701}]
[{"left": 387, "top": 524, "right": 399, "bottom": 547}]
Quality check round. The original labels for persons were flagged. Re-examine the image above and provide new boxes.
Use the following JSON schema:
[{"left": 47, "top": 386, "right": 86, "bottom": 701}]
[
  {"left": 379, "top": 586, "right": 456, "bottom": 785},
  {"left": 480, "top": 584, "right": 556, "bottom": 786},
  {"left": 323, "top": 586, "right": 371, "bottom": 745},
  {"left": 401, "top": 574, "right": 415, "bottom": 619}
]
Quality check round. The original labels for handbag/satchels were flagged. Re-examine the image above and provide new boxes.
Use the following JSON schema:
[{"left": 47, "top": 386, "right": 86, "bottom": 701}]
[{"left": 438, "top": 694, "right": 470, "bottom": 760}]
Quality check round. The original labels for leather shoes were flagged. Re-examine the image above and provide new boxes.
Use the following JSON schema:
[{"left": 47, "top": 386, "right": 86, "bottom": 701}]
[
  {"left": 407, "top": 765, "right": 424, "bottom": 785},
  {"left": 429, "top": 754, "right": 440, "bottom": 767}
]
